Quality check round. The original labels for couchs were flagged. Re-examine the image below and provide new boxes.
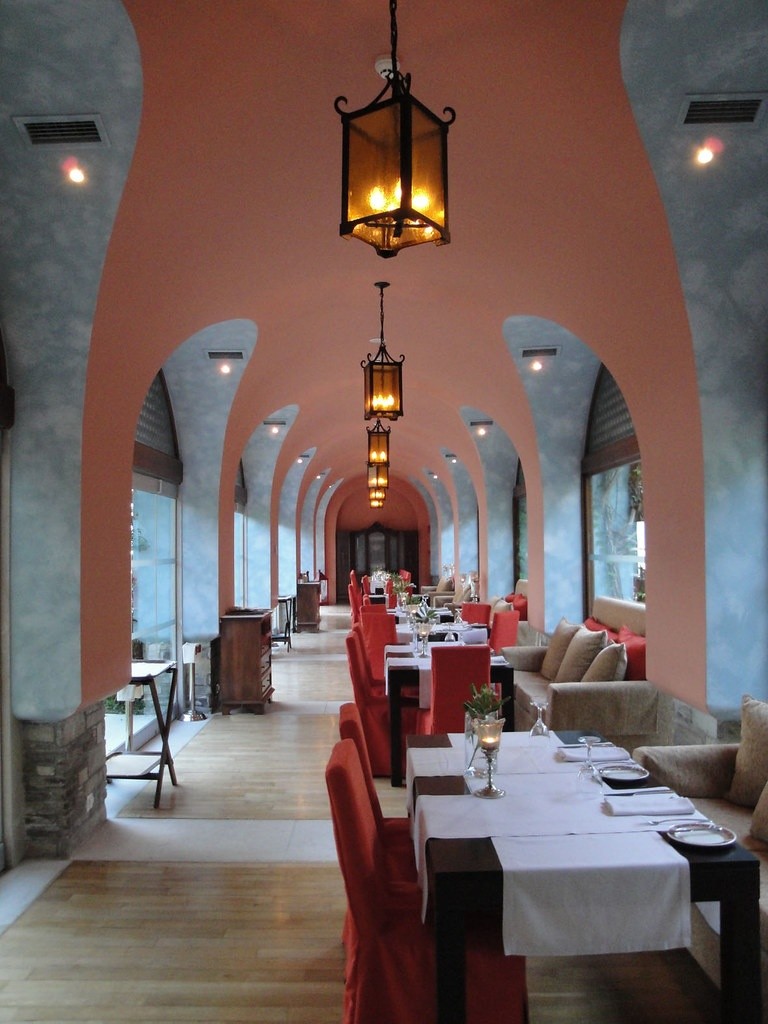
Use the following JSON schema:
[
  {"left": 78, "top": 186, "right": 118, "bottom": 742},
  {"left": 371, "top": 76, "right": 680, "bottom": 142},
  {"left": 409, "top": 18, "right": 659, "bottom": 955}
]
[
  {"left": 421, "top": 576, "right": 462, "bottom": 615},
  {"left": 502, "top": 597, "right": 659, "bottom": 747},
  {"left": 434, "top": 594, "right": 477, "bottom": 611},
  {"left": 488, "top": 579, "right": 529, "bottom": 628},
  {"left": 633, "top": 744, "right": 768, "bottom": 972}
]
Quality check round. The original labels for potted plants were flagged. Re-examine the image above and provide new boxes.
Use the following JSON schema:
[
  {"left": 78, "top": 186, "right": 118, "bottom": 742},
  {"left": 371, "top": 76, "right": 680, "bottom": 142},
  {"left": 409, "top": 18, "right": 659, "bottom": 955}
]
[
  {"left": 462, "top": 682, "right": 512, "bottom": 779},
  {"left": 410, "top": 605, "right": 437, "bottom": 651},
  {"left": 393, "top": 584, "right": 406, "bottom": 611}
]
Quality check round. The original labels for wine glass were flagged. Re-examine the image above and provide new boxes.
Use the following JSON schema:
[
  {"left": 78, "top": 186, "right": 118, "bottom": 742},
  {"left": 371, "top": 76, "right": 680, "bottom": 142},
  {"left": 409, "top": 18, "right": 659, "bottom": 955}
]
[
  {"left": 399, "top": 591, "right": 431, "bottom": 615},
  {"left": 575, "top": 736, "right": 602, "bottom": 800},
  {"left": 529, "top": 700, "right": 551, "bottom": 752},
  {"left": 453, "top": 608, "right": 463, "bottom": 627},
  {"left": 443, "top": 622, "right": 454, "bottom": 644},
  {"left": 418, "top": 623, "right": 432, "bottom": 658},
  {"left": 472, "top": 719, "right": 506, "bottom": 799}
]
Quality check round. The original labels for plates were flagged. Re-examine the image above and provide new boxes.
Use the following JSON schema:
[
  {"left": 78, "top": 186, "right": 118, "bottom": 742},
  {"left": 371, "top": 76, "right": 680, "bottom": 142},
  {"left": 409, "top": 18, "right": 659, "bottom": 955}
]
[
  {"left": 667, "top": 822, "right": 736, "bottom": 846},
  {"left": 599, "top": 765, "right": 650, "bottom": 787}
]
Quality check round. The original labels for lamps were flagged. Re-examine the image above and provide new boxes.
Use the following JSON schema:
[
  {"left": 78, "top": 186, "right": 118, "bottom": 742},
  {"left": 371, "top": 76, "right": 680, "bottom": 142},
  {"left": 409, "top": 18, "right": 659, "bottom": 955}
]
[
  {"left": 366, "top": 418, "right": 392, "bottom": 466},
  {"left": 368, "top": 489, "right": 386, "bottom": 500},
  {"left": 370, "top": 500, "right": 384, "bottom": 509},
  {"left": 367, "top": 463, "right": 389, "bottom": 488},
  {"left": 359, "top": 280, "right": 406, "bottom": 422},
  {"left": 333, "top": 0, "right": 457, "bottom": 260}
]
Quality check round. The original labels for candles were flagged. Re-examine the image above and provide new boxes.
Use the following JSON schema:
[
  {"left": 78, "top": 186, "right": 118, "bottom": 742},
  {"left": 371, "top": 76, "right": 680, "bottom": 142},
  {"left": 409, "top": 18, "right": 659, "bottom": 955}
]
[
  {"left": 475, "top": 718, "right": 507, "bottom": 799},
  {"left": 415, "top": 623, "right": 434, "bottom": 659}
]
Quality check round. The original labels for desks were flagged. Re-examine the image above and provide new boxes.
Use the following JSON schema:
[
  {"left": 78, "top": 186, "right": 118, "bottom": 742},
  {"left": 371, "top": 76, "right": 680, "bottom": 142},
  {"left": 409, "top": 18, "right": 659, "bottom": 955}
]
[
  {"left": 387, "top": 608, "right": 448, "bottom": 624},
  {"left": 368, "top": 594, "right": 431, "bottom": 606},
  {"left": 396, "top": 622, "right": 460, "bottom": 643},
  {"left": 404, "top": 727, "right": 761, "bottom": 1024},
  {"left": 383, "top": 641, "right": 514, "bottom": 787}
]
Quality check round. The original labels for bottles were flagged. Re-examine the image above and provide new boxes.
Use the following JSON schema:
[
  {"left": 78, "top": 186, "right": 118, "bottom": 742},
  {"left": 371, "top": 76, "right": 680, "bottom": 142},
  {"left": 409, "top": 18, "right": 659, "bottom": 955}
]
[{"left": 298, "top": 572, "right": 308, "bottom": 583}]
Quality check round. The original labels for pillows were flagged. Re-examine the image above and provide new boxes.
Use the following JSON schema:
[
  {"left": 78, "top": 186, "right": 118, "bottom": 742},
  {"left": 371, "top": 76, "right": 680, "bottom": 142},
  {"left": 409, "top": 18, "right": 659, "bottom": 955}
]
[
  {"left": 490, "top": 596, "right": 502, "bottom": 609},
  {"left": 514, "top": 598, "right": 527, "bottom": 620},
  {"left": 583, "top": 614, "right": 619, "bottom": 643},
  {"left": 506, "top": 592, "right": 515, "bottom": 602},
  {"left": 749, "top": 780, "right": 768, "bottom": 844},
  {"left": 436, "top": 578, "right": 452, "bottom": 592},
  {"left": 554, "top": 627, "right": 608, "bottom": 682},
  {"left": 617, "top": 623, "right": 646, "bottom": 681},
  {"left": 512, "top": 594, "right": 523, "bottom": 605},
  {"left": 463, "top": 589, "right": 472, "bottom": 602},
  {"left": 453, "top": 585, "right": 470, "bottom": 601},
  {"left": 581, "top": 641, "right": 627, "bottom": 681},
  {"left": 723, "top": 693, "right": 768, "bottom": 806},
  {"left": 541, "top": 615, "right": 582, "bottom": 680},
  {"left": 491, "top": 600, "right": 514, "bottom": 622}
]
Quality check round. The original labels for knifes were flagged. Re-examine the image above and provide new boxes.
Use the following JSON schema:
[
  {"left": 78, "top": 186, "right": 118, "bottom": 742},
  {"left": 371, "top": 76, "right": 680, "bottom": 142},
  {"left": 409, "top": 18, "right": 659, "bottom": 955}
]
[
  {"left": 557, "top": 743, "right": 614, "bottom": 748},
  {"left": 604, "top": 789, "right": 674, "bottom": 797}
]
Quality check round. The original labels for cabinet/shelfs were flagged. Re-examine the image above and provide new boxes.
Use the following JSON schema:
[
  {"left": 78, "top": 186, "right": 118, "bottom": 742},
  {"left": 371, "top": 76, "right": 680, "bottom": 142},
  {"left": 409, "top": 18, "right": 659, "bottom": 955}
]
[
  {"left": 220, "top": 609, "right": 276, "bottom": 716},
  {"left": 106, "top": 660, "right": 180, "bottom": 808},
  {"left": 297, "top": 582, "right": 322, "bottom": 633},
  {"left": 270, "top": 595, "right": 294, "bottom": 653}
]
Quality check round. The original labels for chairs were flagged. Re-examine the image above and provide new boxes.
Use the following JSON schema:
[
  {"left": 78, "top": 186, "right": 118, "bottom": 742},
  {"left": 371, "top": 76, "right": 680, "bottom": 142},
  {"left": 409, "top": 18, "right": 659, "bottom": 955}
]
[
  {"left": 362, "top": 575, "right": 372, "bottom": 595},
  {"left": 344, "top": 632, "right": 419, "bottom": 775},
  {"left": 326, "top": 738, "right": 530, "bottom": 1024},
  {"left": 399, "top": 570, "right": 413, "bottom": 583},
  {"left": 461, "top": 603, "right": 491, "bottom": 629},
  {"left": 415, "top": 646, "right": 496, "bottom": 737},
  {"left": 383, "top": 580, "right": 392, "bottom": 595},
  {"left": 387, "top": 585, "right": 413, "bottom": 609},
  {"left": 362, "top": 594, "right": 371, "bottom": 606},
  {"left": 361, "top": 612, "right": 396, "bottom": 679},
  {"left": 489, "top": 611, "right": 520, "bottom": 699},
  {"left": 349, "top": 570, "right": 361, "bottom": 597},
  {"left": 349, "top": 584, "right": 361, "bottom": 623},
  {"left": 358, "top": 603, "right": 386, "bottom": 615},
  {"left": 352, "top": 622, "right": 387, "bottom": 685},
  {"left": 340, "top": 702, "right": 409, "bottom": 836}
]
